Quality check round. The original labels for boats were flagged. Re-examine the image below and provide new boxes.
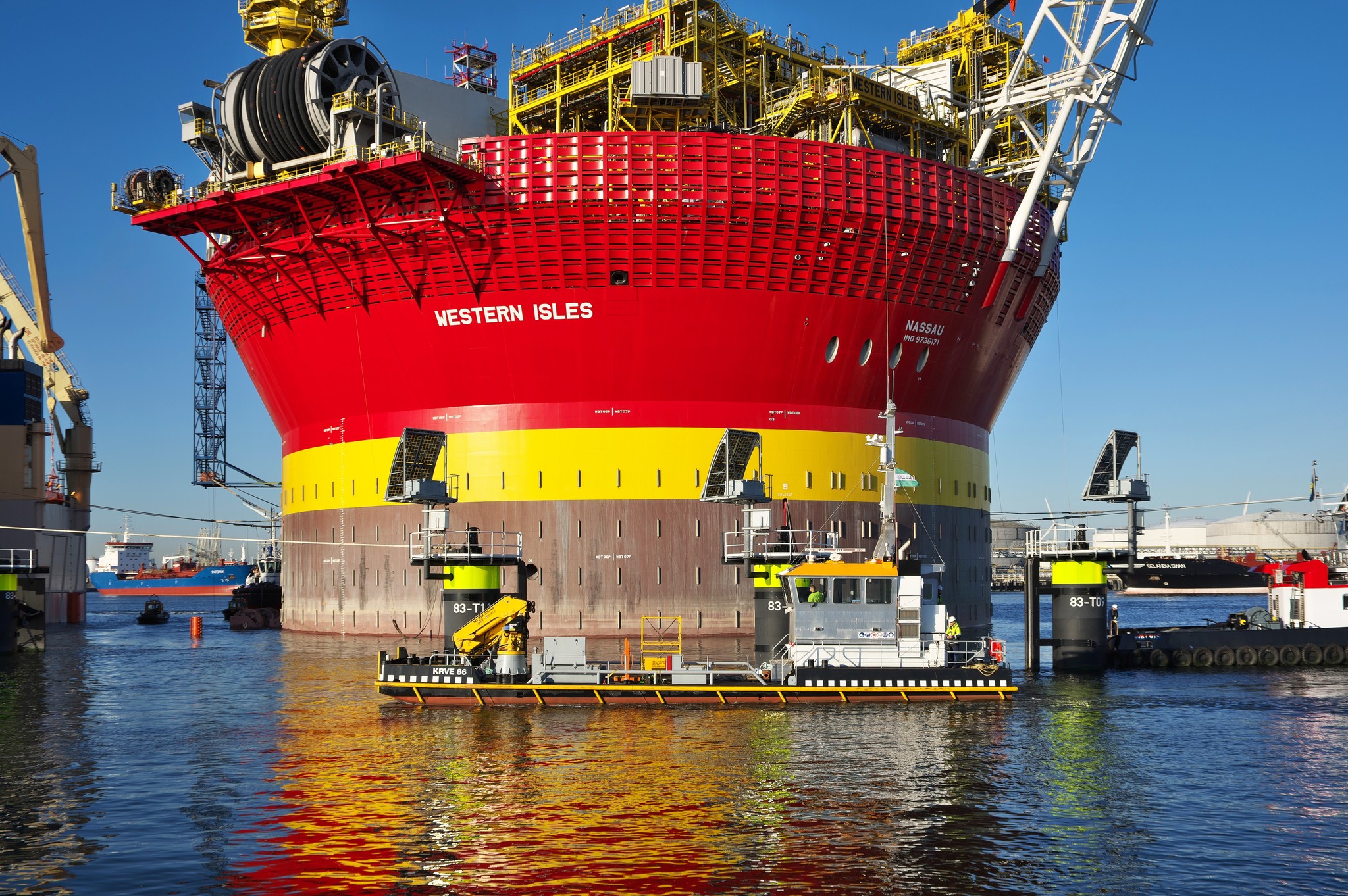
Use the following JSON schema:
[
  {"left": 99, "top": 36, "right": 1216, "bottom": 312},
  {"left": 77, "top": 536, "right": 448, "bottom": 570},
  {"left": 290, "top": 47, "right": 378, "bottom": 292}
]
[
  {"left": 244, "top": 504, "right": 282, "bottom": 586},
  {"left": 1105, "top": 548, "right": 1348, "bottom": 675},
  {"left": 86, "top": 514, "right": 258, "bottom": 597}
]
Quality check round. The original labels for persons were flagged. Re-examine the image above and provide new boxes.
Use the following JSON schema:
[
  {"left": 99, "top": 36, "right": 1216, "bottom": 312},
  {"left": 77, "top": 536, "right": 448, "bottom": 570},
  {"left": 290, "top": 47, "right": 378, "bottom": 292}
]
[
  {"left": 1108, "top": 604, "right": 1118, "bottom": 635},
  {"left": 946, "top": 616, "right": 962, "bottom": 669},
  {"left": 268, "top": 565, "right": 273, "bottom": 571},
  {"left": 808, "top": 586, "right": 824, "bottom": 602}
]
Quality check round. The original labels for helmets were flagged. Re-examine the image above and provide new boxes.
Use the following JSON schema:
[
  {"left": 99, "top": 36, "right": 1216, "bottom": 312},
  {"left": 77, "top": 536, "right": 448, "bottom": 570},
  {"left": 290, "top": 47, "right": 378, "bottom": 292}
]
[
  {"left": 938, "top": 585, "right": 943, "bottom": 590},
  {"left": 949, "top": 616, "right": 956, "bottom": 622},
  {"left": 1112, "top": 604, "right": 1118, "bottom": 609}
]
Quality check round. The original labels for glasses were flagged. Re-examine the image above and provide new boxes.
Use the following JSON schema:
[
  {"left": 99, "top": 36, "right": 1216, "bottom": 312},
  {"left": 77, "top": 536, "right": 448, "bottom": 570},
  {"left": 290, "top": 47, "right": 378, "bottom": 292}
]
[{"left": 951, "top": 622, "right": 953, "bottom": 623}]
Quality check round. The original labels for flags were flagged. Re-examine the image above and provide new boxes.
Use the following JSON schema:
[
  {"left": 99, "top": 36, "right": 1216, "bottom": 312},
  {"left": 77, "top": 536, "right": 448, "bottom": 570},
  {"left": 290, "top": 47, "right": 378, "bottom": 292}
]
[{"left": 1309, "top": 469, "right": 1316, "bottom": 502}]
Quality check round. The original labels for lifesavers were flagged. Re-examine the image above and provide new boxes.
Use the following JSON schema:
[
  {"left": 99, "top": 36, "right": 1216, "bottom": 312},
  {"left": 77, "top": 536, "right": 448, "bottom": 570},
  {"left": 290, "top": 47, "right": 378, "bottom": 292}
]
[
  {"left": 1102, "top": 649, "right": 1125, "bottom": 670},
  {"left": 1279, "top": 643, "right": 1301, "bottom": 667},
  {"left": 1193, "top": 647, "right": 1214, "bottom": 668},
  {"left": 1324, "top": 644, "right": 1346, "bottom": 668},
  {"left": 1124, "top": 649, "right": 1147, "bottom": 669},
  {"left": 1148, "top": 648, "right": 1169, "bottom": 669},
  {"left": 1258, "top": 644, "right": 1280, "bottom": 668},
  {"left": 1214, "top": 646, "right": 1235, "bottom": 668},
  {"left": 1236, "top": 645, "right": 1258, "bottom": 668},
  {"left": 995, "top": 642, "right": 1003, "bottom": 663},
  {"left": 1169, "top": 648, "right": 1193, "bottom": 669},
  {"left": 1301, "top": 643, "right": 1323, "bottom": 668}
]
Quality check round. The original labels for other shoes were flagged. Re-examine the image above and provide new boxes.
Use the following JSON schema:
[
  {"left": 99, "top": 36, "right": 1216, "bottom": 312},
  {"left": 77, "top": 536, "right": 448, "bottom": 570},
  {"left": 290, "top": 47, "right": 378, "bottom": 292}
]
[{"left": 952, "top": 666, "right": 957, "bottom": 669}]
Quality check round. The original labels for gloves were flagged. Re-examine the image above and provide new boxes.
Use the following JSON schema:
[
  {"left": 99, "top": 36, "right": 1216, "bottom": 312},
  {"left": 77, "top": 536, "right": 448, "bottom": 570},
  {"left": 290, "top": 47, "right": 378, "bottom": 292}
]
[
  {"left": 1113, "top": 620, "right": 1118, "bottom": 624},
  {"left": 1113, "top": 616, "right": 1116, "bottom": 619}
]
